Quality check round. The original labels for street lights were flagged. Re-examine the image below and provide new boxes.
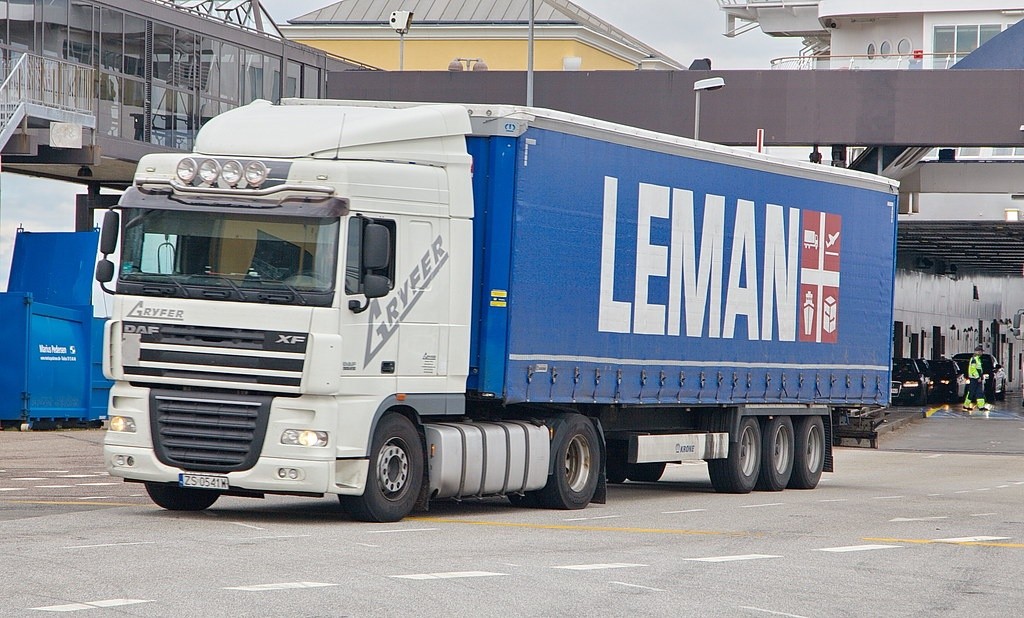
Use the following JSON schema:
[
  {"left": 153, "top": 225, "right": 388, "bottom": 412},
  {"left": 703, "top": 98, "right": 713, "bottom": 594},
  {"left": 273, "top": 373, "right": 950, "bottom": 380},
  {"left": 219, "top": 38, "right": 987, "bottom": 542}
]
[{"left": 692, "top": 76, "right": 727, "bottom": 140}]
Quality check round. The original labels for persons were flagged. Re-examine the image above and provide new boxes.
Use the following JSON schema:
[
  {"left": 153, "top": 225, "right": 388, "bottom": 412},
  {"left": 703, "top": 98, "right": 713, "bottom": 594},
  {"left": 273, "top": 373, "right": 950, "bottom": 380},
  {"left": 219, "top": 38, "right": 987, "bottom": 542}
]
[{"left": 962, "top": 346, "right": 989, "bottom": 411}]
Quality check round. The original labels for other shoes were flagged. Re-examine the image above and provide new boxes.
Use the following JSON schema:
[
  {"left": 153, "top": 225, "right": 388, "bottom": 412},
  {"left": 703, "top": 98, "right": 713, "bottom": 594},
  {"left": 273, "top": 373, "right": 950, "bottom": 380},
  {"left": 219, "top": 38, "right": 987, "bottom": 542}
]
[
  {"left": 979, "top": 407, "right": 988, "bottom": 411},
  {"left": 962, "top": 406, "right": 973, "bottom": 411}
]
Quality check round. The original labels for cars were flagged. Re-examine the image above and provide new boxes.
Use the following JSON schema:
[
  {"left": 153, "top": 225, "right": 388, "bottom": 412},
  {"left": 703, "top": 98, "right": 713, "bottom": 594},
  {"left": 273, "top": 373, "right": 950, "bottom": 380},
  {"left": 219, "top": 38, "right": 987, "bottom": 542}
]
[
  {"left": 927, "top": 358, "right": 967, "bottom": 403},
  {"left": 953, "top": 353, "right": 1008, "bottom": 402},
  {"left": 888, "top": 358, "right": 929, "bottom": 406}
]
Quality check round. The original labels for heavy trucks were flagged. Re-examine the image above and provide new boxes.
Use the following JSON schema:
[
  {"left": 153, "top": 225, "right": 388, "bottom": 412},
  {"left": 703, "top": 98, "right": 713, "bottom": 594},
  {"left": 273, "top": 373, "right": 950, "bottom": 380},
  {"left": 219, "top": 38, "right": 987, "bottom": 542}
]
[{"left": 91, "top": 96, "right": 903, "bottom": 523}]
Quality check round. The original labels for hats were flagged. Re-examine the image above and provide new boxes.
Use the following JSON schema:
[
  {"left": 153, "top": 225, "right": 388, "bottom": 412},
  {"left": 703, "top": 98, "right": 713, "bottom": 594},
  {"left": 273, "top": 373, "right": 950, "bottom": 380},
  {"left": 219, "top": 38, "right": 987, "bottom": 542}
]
[{"left": 975, "top": 347, "right": 985, "bottom": 351}]
